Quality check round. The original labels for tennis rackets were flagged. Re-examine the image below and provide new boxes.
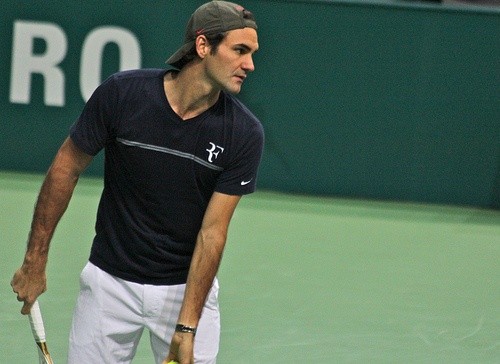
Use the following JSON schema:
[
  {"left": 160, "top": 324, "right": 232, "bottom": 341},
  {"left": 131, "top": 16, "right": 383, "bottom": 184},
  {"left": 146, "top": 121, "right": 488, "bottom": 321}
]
[{"left": 28, "top": 299, "right": 52, "bottom": 364}]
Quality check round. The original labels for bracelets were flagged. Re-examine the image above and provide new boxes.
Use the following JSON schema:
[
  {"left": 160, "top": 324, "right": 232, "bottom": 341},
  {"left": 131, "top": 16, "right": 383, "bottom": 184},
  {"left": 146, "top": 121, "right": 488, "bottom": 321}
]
[{"left": 176, "top": 322, "right": 198, "bottom": 338}]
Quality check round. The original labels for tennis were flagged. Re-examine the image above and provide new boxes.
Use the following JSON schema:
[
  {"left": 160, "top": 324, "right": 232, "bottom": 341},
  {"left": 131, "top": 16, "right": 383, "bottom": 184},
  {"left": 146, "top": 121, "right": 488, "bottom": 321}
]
[{"left": 167, "top": 360, "right": 178, "bottom": 364}]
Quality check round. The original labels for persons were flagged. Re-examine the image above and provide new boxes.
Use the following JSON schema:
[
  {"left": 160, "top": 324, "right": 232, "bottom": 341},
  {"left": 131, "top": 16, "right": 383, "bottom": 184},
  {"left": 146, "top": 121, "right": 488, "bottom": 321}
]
[{"left": 11, "top": 1, "right": 263, "bottom": 364}]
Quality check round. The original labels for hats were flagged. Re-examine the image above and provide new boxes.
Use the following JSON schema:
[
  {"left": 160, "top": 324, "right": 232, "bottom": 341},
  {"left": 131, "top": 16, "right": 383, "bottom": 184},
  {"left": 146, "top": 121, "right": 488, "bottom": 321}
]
[{"left": 164, "top": 0, "right": 258, "bottom": 66}]
